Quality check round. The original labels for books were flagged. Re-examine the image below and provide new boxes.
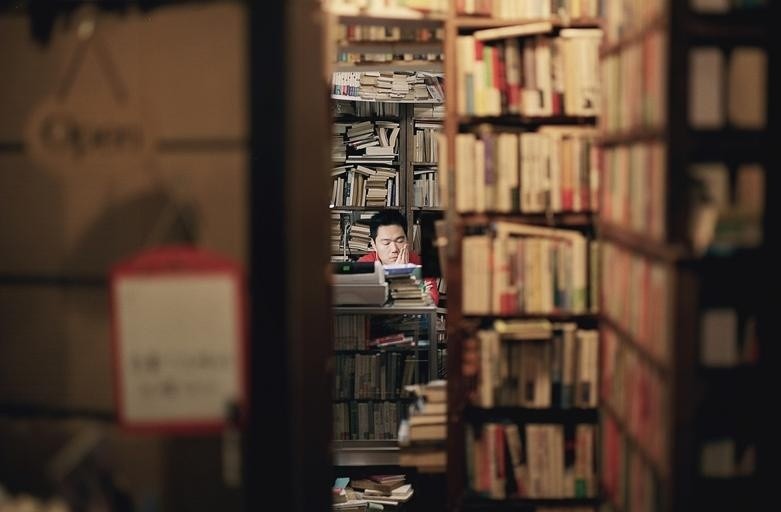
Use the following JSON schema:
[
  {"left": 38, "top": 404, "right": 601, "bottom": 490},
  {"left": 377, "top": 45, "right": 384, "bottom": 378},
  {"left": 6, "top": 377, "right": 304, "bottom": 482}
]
[
  {"left": 461, "top": 222, "right": 598, "bottom": 497},
  {"left": 401, "top": 452, "right": 448, "bottom": 466},
  {"left": 331, "top": 473, "right": 414, "bottom": 511},
  {"left": 332, "top": 273, "right": 447, "bottom": 440},
  {"left": 455, "top": 23, "right": 602, "bottom": 212},
  {"left": 331, "top": 70, "right": 445, "bottom": 263},
  {"left": 456, "top": 0, "right": 601, "bottom": 18},
  {"left": 604, "top": 0, "right": 766, "bottom": 510},
  {"left": 338, "top": 23, "right": 445, "bottom": 63}
]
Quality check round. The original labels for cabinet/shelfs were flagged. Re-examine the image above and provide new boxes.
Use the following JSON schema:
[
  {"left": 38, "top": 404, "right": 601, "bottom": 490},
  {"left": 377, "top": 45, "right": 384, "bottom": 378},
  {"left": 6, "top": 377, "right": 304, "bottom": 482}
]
[
  {"left": 336, "top": 14, "right": 446, "bottom": 63},
  {"left": 329, "top": 306, "right": 449, "bottom": 466},
  {"left": 445, "top": 2, "right": 779, "bottom": 511},
  {"left": 333, "top": 64, "right": 445, "bottom": 262},
  {"left": 0, "top": 1, "right": 333, "bottom": 511}
]
